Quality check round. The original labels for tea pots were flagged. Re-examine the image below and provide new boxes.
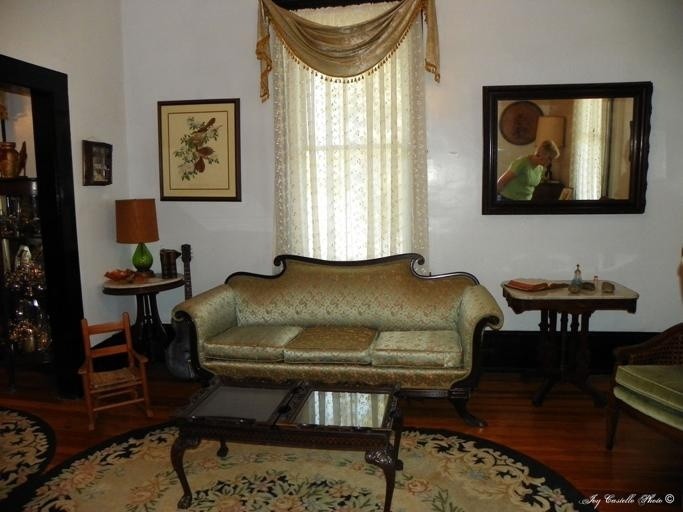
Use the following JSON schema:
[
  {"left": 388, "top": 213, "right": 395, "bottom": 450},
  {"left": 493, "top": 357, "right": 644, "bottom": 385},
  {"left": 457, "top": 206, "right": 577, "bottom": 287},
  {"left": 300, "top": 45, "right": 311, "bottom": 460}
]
[{"left": 159, "top": 248, "right": 181, "bottom": 279}]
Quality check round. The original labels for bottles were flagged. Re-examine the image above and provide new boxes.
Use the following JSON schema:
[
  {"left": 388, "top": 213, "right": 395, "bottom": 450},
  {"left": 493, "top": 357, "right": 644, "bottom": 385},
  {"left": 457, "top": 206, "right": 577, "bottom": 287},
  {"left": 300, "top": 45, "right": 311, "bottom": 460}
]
[
  {"left": 571, "top": 263, "right": 583, "bottom": 288},
  {"left": 0, "top": 195, "right": 52, "bottom": 355},
  {"left": 593, "top": 275, "right": 598, "bottom": 288},
  {"left": 0, "top": 141, "right": 22, "bottom": 178}
]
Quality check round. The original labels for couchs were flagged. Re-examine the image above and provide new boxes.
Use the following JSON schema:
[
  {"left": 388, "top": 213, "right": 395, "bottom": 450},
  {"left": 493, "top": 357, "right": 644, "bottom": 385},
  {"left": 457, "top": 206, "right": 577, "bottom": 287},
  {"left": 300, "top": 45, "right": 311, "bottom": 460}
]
[{"left": 164, "top": 252, "right": 505, "bottom": 430}]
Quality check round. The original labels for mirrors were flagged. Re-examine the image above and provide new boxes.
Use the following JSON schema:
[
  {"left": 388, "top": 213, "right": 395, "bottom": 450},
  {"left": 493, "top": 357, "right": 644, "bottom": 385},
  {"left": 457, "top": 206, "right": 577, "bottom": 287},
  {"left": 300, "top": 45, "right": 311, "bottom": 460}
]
[{"left": 480, "top": 80, "right": 654, "bottom": 216}]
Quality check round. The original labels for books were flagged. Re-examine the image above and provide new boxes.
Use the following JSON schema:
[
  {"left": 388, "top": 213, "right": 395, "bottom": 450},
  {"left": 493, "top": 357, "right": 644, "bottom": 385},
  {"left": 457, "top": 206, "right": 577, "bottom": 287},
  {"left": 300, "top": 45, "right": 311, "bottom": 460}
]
[{"left": 506, "top": 277, "right": 570, "bottom": 291}]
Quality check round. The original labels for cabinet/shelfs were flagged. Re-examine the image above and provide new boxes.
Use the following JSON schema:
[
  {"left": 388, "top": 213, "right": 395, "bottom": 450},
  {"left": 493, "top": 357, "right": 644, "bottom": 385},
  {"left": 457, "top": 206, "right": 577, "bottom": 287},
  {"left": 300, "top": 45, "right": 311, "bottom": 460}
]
[
  {"left": 532, "top": 182, "right": 564, "bottom": 200},
  {"left": 0, "top": 176, "right": 58, "bottom": 376}
]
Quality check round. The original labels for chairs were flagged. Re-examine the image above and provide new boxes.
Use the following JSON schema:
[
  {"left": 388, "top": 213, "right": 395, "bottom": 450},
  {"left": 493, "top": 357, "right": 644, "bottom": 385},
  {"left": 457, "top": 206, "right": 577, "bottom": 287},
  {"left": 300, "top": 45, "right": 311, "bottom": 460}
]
[
  {"left": 602, "top": 321, "right": 682, "bottom": 479},
  {"left": 76, "top": 310, "right": 155, "bottom": 434}
]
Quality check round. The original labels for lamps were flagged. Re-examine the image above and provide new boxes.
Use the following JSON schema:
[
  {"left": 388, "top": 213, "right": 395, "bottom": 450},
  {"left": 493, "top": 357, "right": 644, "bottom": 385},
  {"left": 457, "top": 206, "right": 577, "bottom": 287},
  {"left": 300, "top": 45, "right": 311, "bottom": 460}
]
[
  {"left": 535, "top": 116, "right": 566, "bottom": 183},
  {"left": 113, "top": 197, "right": 159, "bottom": 278}
]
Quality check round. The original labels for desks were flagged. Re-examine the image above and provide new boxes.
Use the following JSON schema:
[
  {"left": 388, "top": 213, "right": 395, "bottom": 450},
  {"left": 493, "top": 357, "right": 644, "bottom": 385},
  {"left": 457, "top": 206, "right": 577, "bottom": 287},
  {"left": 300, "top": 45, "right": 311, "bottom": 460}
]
[
  {"left": 500, "top": 279, "right": 640, "bottom": 410},
  {"left": 100, "top": 274, "right": 185, "bottom": 364}
]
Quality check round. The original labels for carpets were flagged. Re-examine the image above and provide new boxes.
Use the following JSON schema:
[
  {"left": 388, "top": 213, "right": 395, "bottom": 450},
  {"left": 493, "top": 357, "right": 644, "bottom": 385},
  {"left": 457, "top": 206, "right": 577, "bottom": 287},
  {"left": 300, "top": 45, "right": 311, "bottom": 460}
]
[
  {"left": 0, "top": 413, "right": 601, "bottom": 512},
  {"left": 0, "top": 404, "right": 57, "bottom": 511}
]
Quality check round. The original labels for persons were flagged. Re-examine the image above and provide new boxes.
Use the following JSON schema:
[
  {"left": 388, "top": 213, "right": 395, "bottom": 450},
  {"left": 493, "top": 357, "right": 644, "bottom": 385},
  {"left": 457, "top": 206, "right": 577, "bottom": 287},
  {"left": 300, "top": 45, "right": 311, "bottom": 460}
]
[{"left": 496, "top": 139, "right": 560, "bottom": 200}]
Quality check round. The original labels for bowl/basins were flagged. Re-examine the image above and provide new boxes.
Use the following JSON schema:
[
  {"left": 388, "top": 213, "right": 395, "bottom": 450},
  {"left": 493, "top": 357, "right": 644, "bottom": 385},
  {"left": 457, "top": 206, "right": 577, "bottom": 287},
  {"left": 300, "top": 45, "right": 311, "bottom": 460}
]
[{"left": 103, "top": 266, "right": 136, "bottom": 283}]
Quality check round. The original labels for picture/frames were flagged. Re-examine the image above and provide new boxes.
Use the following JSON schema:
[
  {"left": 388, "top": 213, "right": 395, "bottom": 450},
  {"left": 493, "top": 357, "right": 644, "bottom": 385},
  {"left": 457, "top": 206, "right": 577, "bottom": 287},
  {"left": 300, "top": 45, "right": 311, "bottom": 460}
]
[
  {"left": 499, "top": 100, "right": 544, "bottom": 145},
  {"left": 557, "top": 186, "right": 573, "bottom": 200},
  {"left": 80, "top": 138, "right": 113, "bottom": 188},
  {"left": 155, "top": 97, "right": 242, "bottom": 204}
]
